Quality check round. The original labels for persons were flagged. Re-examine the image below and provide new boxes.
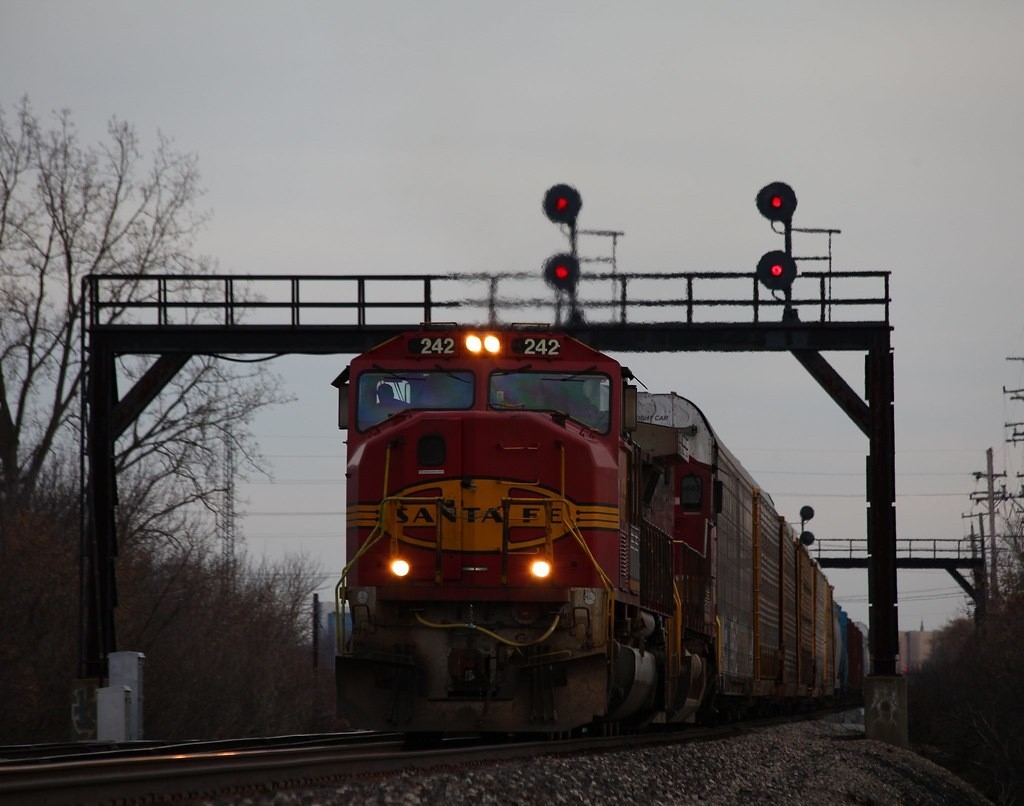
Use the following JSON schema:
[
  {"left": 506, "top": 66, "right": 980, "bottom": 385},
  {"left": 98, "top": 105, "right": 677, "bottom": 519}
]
[{"left": 368, "top": 383, "right": 411, "bottom": 428}]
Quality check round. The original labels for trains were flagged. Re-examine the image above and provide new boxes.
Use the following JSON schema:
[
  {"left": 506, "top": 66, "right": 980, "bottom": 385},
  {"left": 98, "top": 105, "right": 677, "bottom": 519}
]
[{"left": 332, "top": 317, "right": 869, "bottom": 736}]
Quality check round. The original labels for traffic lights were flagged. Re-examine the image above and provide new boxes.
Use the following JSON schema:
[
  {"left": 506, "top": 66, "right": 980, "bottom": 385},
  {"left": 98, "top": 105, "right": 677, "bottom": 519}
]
[
  {"left": 755, "top": 181, "right": 798, "bottom": 223},
  {"left": 543, "top": 183, "right": 584, "bottom": 223},
  {"left": 542, "top": 253, "right": 581, "bottom": 291},
  {"left": 757, "top": 250, "right": 798, "bottom": 290}
]
